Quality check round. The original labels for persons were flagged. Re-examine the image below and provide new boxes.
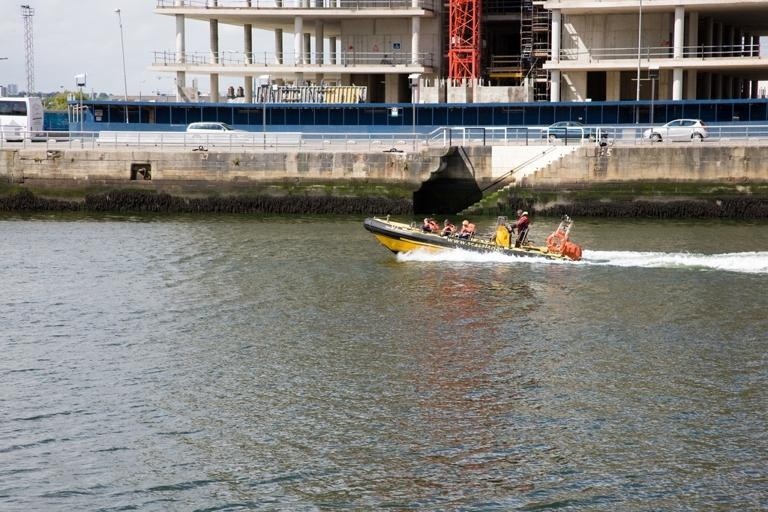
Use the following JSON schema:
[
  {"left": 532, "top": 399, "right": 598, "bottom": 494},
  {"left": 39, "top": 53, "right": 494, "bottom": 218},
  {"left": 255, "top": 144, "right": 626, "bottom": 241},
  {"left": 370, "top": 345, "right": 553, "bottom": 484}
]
[
  {"left": 419, "top": 216, "right": 433, "bottom": 234},
  {"left": 440, "top": 219, "right": 456, "bottom": 236},
  {"left": 458, "top": 219, "right": 476, "bottom": 239},
  {"left": 508, "top": 209, "right": 530, "bottom": 248},
  {"left": 428, "top": 217, "right": 440, "bottom": 235}
]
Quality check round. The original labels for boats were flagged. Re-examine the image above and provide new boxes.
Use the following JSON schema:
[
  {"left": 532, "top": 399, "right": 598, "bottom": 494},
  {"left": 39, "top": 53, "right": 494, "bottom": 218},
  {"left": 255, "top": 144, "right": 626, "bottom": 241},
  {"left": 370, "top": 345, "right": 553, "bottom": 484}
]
[{"left": 363, "top": 212, "right": 583, "bottom": 264}]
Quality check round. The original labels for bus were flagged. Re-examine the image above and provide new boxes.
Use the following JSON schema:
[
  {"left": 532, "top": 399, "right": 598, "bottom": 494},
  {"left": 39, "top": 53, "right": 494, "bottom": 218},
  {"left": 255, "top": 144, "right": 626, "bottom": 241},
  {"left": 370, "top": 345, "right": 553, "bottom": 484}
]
[{"left": 0, "top": 96, "right": 44, "bottom": 141}]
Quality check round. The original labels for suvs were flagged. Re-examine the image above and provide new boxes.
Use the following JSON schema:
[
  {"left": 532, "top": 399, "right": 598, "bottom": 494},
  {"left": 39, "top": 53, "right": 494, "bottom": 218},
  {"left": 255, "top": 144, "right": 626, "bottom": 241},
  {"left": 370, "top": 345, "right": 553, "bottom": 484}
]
[{"left": 186, "top": 122, "right": 252, "bottom": 145}]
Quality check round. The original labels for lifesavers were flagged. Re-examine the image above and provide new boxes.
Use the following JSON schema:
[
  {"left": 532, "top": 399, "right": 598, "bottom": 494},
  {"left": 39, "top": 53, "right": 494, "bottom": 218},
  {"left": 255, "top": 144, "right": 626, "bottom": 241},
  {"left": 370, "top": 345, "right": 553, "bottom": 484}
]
[{"left": 546, "top": 231, "right": 569, "bottom": 252}]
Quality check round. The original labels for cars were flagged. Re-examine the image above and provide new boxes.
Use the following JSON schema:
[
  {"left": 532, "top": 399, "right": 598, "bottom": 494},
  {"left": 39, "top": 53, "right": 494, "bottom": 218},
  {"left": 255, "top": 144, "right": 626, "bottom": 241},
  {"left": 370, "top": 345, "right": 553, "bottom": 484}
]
[
  {"left": 541, "top": 120, "right": 608, "bottom": 143},
  {"left": 644, "top": 118, "right": 710, "bottom": 143}
]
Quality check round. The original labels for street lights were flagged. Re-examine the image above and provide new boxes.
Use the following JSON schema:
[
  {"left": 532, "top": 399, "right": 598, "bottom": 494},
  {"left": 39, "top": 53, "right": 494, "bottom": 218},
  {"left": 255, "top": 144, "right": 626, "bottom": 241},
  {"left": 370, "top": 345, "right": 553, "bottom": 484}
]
[
  {"left": 114, "top": 7, "right": 130, "bottom": 124},
  {"left": 76, "top": 72, "right": 87, "bottom": 149}
]
